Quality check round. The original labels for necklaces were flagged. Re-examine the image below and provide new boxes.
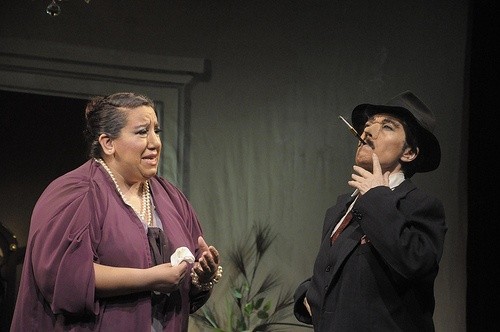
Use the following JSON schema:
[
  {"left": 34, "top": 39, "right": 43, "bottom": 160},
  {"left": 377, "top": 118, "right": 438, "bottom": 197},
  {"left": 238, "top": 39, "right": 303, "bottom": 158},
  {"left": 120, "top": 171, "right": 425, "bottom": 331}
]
[{"left": 95, "top": 156, "right": 151, "bottom": 226}]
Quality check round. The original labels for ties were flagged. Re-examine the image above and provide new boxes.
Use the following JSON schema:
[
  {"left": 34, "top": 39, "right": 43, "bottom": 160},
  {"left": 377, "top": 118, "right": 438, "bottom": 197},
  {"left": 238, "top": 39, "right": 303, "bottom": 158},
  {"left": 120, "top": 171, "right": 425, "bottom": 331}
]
[{"left": 331, "top": 211, "right": 353, "bottom": 244}]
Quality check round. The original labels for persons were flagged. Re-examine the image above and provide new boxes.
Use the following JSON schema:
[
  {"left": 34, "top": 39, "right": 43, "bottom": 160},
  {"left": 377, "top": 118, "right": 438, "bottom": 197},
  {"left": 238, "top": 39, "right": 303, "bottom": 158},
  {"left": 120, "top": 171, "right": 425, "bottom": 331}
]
[
  {"left": 10, "top": 92, "right": 220, "bottom": 332},
  {"left": 293, "top": 89, "right": 449, "bottom": 332}
]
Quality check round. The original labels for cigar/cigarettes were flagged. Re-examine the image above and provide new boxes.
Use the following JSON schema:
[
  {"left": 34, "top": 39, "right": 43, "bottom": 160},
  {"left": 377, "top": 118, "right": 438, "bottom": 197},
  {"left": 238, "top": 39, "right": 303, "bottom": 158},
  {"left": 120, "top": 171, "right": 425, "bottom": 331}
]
[{"left": 339, "top": 115, "right": 367, "bottom": 145}]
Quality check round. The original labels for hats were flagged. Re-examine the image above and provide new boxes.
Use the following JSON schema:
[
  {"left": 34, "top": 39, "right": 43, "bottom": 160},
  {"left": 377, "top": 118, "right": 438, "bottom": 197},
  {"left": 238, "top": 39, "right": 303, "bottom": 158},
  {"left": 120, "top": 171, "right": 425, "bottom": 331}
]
[{"left": 351, "top": 90, "right": 440, "bottom": 173}]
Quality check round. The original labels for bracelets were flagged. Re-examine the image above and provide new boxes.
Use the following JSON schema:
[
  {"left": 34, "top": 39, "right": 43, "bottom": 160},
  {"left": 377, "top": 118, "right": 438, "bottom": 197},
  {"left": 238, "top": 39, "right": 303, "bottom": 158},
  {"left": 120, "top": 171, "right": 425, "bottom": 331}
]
[{"left": 192, "top": 266, "right": 222, "bottom": 289}]
